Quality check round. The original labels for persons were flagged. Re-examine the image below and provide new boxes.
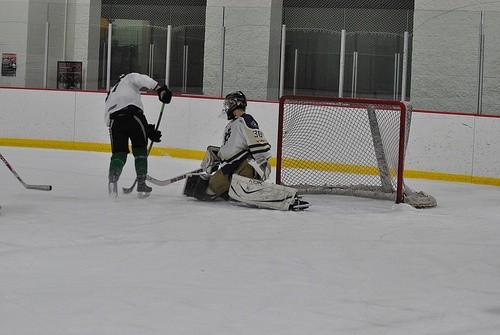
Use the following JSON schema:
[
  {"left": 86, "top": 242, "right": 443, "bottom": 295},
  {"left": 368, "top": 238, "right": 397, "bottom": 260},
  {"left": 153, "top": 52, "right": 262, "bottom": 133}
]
[
  {"left": 197, "top": 91, "right": 272, "bottom": 202},
  {"left": 104, "top": 72, "right": 172, "bottom": 199},
  {"left": 58, "top": 67, "right": 81, "bottom": 90},
  {"left": 5, "top": 56, "right": 16, "bottom": 74}
]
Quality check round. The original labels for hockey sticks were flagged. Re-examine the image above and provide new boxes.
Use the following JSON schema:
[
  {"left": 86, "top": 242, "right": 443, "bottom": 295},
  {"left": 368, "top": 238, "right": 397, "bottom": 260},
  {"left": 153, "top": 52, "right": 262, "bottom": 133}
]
[
  {"left": 0, "top": 154, "right": 52, "bottom": 191},
  {"left": 123, "top": 103, "right": 165, "bottom": 194},
  {"left": 146, "top": 168, "right": 203, "bottom": 186}
]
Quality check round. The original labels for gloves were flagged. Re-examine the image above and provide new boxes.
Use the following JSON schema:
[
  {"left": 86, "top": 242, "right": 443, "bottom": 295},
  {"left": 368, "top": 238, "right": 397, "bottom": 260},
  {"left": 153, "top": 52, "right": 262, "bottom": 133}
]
[
  {"left": 147, "top": 124, "right": 163, "bottom": 143},
  {"left": 156, "top": 84, "right": 172, "bottom": 104}
]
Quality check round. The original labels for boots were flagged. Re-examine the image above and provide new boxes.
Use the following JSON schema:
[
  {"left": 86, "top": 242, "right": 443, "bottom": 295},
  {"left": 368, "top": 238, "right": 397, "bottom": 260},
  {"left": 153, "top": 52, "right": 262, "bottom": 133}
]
[
  {"left": 108, "top": 159, "right": 124, "bottom": 198},
  {"left": 135, "top": 156, "right": 152, "bottom": 192}
]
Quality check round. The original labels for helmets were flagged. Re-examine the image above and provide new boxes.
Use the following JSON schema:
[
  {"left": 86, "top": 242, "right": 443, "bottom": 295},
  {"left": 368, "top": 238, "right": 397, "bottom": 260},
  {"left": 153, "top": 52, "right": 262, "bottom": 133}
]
[
  {"left": 117, "top": 74, "right": 124, "bottom": 80},
  {"left": 224, "top": 91, "right": 247, "bottom": 120}
]
